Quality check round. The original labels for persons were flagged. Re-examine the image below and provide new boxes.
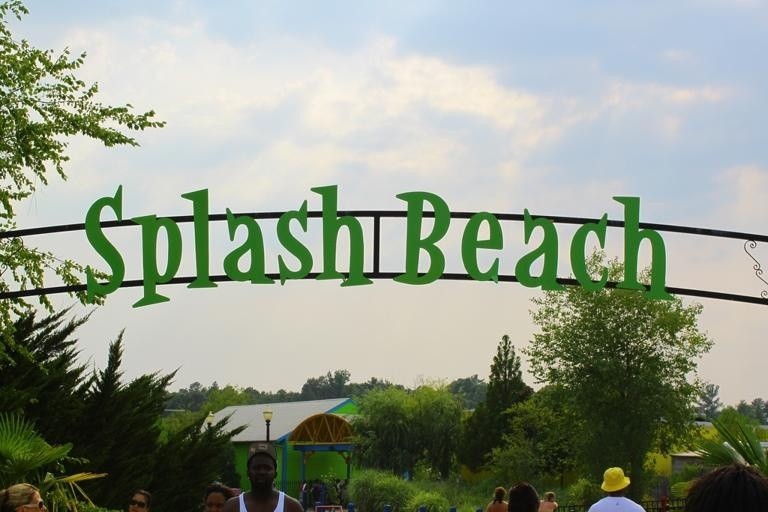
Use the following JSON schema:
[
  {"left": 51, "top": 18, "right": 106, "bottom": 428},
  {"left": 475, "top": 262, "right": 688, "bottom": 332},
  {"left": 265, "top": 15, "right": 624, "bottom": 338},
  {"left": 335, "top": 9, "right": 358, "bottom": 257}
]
[
  {"left": 128, "top": 488, "right": 151, "bottom": 512},
  {"left": 588, "top": 466, "right": 647, "bottom": 512},
  {"left": 684, "top": 464, "right": 768, "bottom": 512},
  {"left": 486, "top": 480, "right": 559, "bottom": 512},
  {"left": 298, "top": 480, "right": 347, "bottom": 510},
  {"left": 0, "top": 483, "right": 47, "bottom": 512},
  {"left": 223, "top": 450, "right": 305, "bottom": 512},
  {"left": 203, "top": 480, "right": 235, "bottom": 512}
]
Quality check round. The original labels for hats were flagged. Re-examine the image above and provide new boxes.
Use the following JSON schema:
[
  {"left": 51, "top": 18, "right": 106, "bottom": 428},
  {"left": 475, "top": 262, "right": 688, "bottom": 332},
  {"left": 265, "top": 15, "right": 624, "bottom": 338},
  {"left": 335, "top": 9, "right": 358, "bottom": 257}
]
[
  {"left": 600, "top": 467, "right": 631, "bottom": 493},
  {"left": 247, "top": 442, "right": 276, "bottom": 461}
]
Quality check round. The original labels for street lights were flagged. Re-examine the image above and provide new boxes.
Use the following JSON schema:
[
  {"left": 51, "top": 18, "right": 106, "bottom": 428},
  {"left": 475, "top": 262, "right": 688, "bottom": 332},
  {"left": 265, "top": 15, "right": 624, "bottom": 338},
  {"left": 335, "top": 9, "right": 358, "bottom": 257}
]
[
  {"left": 204, "top": 409, "right": 214, "bottom": 432},
  {"left": 262, "top": 403, "right": 273, "bottom": 444}
]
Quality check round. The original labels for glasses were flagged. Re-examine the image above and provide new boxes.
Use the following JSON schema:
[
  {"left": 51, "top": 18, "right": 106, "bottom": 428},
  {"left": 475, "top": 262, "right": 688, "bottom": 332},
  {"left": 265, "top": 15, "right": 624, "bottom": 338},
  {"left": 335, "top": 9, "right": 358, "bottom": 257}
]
[
  {"left": 129, "top": 499, "right": 146, "bottom": 509},
  {"left": 23, "top": 502, "right": 44, "bottom": 511}
]
